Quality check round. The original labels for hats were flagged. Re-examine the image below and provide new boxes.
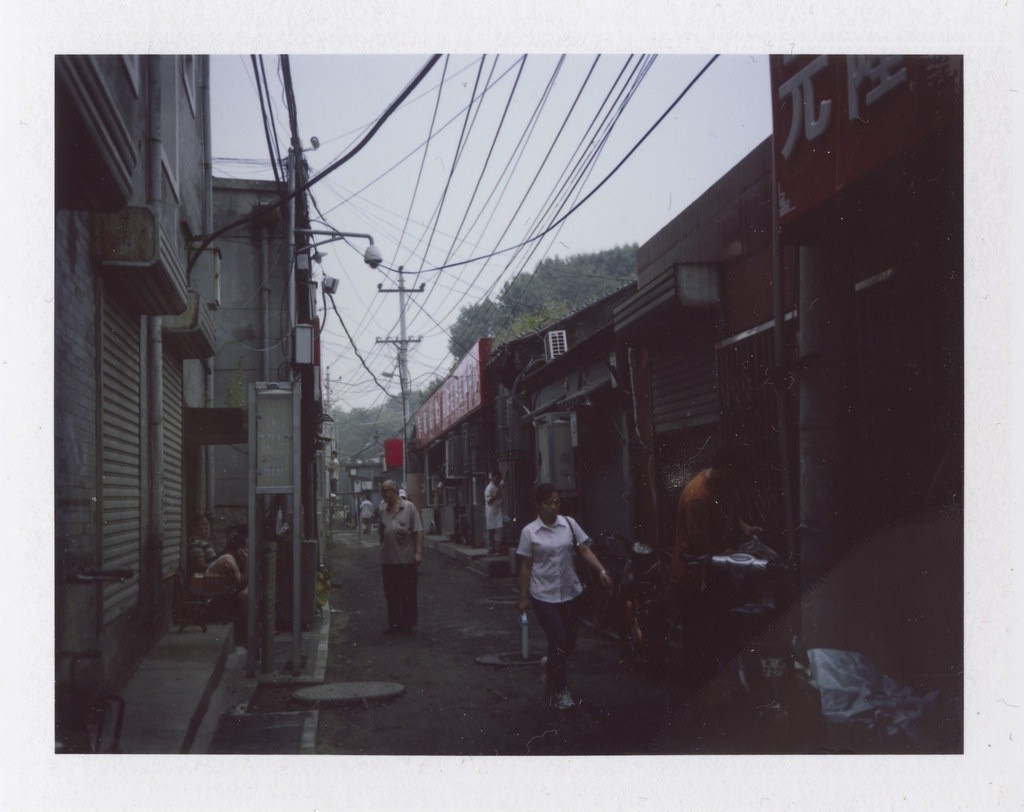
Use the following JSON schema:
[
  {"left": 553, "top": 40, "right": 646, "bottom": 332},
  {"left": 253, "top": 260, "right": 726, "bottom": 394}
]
[{"left": 399, "top": 489, "right": 406, "bottom": 497}]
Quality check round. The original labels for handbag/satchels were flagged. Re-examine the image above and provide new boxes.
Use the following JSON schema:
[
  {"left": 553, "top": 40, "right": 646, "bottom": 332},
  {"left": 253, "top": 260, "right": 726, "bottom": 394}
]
[{"left": 572, "top": 554, "right": 593, "bottom": 583}]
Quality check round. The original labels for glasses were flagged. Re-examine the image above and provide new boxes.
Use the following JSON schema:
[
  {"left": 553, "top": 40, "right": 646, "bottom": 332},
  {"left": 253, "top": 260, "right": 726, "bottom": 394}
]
[{"left": 382, "top": 488, "right": 397, "bottom": 492}]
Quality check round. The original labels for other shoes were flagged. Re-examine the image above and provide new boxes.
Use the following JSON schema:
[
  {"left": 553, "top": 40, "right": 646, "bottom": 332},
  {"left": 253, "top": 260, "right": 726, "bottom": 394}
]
[
  {"left": 382, "top": 626, "right": 398, "bottom": 635},
  {"left": 488, "top": 547, "right": 500, "bottom": 555},
  {"left": 399, "top": 626, "right": 413, "bottom": 635}
]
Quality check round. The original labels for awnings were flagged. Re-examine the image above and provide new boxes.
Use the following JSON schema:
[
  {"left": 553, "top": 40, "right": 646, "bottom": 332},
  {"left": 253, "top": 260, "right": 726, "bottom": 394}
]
[
  {"left": 555, "top": 378, "right": 609, "bottom": 410},
  {"left": 521, "top": 392, "right": 564, "bottom": 424}
]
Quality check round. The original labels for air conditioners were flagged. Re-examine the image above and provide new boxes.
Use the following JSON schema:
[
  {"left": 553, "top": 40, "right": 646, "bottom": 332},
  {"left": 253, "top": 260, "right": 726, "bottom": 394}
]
[{"left": 543, "top": 329, "right": 568, "bottom": 363}]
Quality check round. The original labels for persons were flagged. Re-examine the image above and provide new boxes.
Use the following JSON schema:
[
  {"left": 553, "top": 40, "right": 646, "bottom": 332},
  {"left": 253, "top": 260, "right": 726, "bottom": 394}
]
[
  {"left": 226, "top": 526, "right": 248, "bottom": 574},
  {"left": 378, "top": 479, "right": 423, "bottom": 634},
  {"left": 327, "top": 451, "right": 339, "bottom": 492},
  {"left": 189, "top": 514, "right": 218, "bottom": 573},
  {"left": 671, "top": 442, "right": 764, "bottom": 693},
  {"left": 517, "top": 483, "right": 613, "bottom": 711},
  {"left": 359, "top": 493, "right": 375, "bottom": 535},
  {"left": 204, "top": 537, "right": 248, "bottom": 616},
  {"left": 484, "top": 470, "right": 513, "bottom": 553}
]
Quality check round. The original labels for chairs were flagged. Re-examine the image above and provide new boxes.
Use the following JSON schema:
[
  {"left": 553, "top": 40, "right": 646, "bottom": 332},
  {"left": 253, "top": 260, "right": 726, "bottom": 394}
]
[{"left": 175, "top": 569, "right": 209, "bottom": 633}]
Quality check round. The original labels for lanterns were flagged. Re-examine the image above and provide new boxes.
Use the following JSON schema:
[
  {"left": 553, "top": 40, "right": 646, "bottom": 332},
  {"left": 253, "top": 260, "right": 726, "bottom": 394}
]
[{"left": 384, "top": 438, "right": 403, "bottom": 468}]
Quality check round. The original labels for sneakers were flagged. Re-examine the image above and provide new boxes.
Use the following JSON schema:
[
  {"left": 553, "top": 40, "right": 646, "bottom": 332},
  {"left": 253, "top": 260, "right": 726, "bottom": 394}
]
[
  {"left": 541, "top": 657, "right": 552, "bottom": 685},
  {"left": 549, "top": 689, "right": 576, "bottom": 710}
]
